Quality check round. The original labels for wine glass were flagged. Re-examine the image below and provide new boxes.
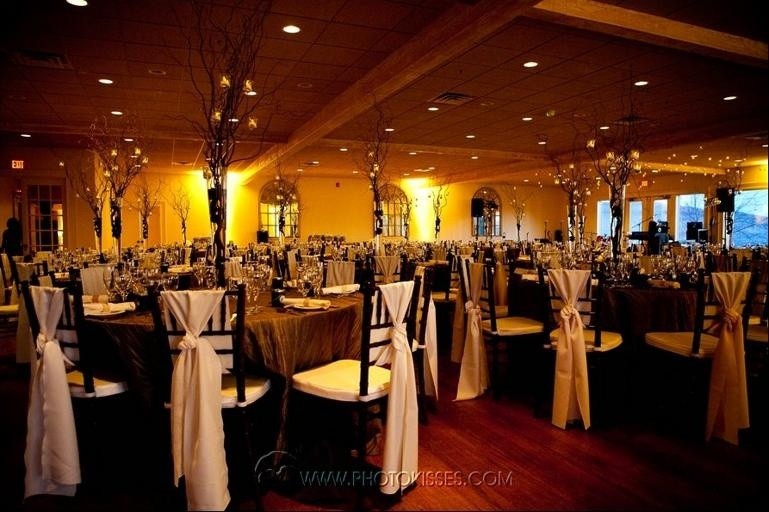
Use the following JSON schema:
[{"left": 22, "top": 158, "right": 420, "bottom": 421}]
[
  {"left": 229, "top": 261, "right": 271, "bottom": 315},
  {"left": 192, "top": 262, "right": 206, "bottom": 290},
  {"left": 102, "top": 269, "right": 132, "bottom": 303},
  {"left": 295, "top": 261, "right": 324, "bottom": 299}
]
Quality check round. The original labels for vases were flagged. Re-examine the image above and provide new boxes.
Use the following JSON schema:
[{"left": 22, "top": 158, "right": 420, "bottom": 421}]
[
  {"left": 278, "top": 212, "right": 285, "bottom": 247},
  {"left": 91, "top": 196, "right": 149, "bottom": 263},
  {"left": 202, "top": 170, "right": 232, "bottom": 287},
  {"left": 567, "top": 214, "right": 587, "bottom": 255}
]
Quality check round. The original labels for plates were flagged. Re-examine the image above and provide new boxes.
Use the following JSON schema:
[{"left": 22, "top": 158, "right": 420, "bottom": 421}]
[
  {"left": 86, "top": 309, "right": 125, "bottom": 317},
  {"left": 293, "top": 304, "right": 322, "bottom": 310}
]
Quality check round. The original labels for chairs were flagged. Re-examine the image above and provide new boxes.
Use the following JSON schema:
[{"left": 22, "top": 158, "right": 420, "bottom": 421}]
[{"left": 0, "top": 235, "right": 769, "bottom": 511}]
